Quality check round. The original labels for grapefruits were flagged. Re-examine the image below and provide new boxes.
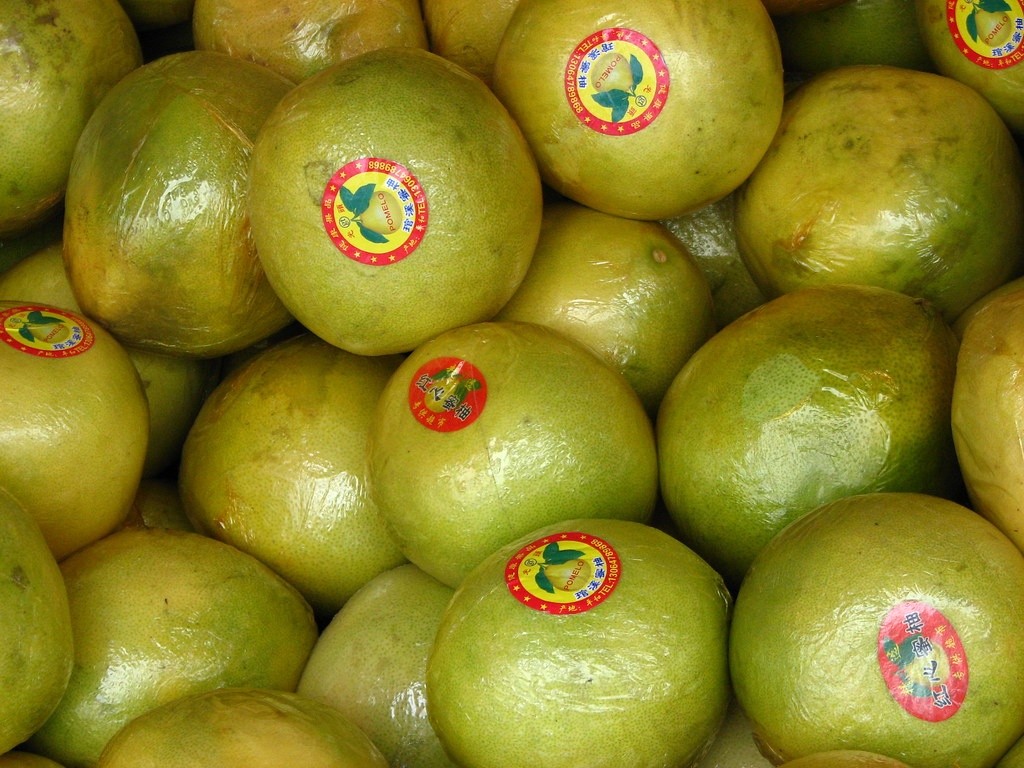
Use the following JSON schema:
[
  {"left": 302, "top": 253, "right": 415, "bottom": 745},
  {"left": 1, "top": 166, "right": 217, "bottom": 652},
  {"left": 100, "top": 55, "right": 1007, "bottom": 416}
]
[{"left": 0, "top": 0, "right": 1024, "bottom": 768}]
